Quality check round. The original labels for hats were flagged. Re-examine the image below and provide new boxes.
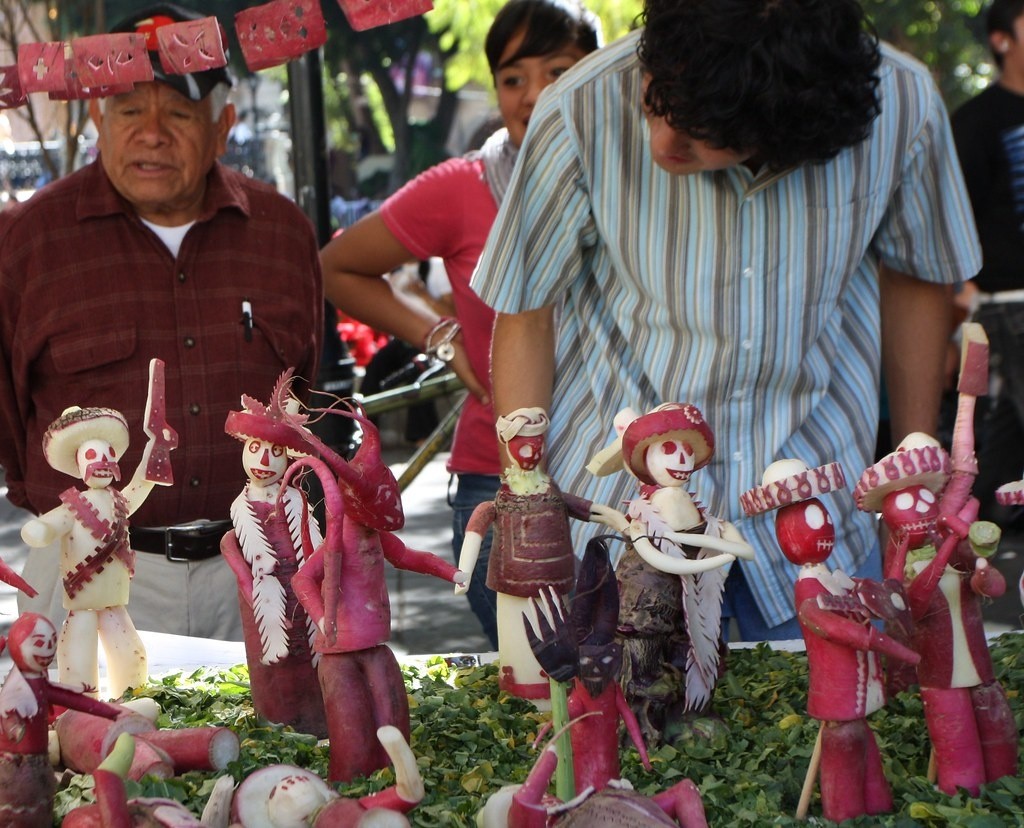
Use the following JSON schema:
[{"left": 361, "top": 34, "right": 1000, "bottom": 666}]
[{"left": 103, "top": 3, "right": 233, "bottom": 102}]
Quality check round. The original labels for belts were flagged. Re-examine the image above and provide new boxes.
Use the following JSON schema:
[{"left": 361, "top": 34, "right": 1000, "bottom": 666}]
[{"left": 128, "top": 521, "right": 232, "bottom": 564}]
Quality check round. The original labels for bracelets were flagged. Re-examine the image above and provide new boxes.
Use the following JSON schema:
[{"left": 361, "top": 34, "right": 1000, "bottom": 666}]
[{"left": 423, "top": 314, "right": 461, "bottom": 362}]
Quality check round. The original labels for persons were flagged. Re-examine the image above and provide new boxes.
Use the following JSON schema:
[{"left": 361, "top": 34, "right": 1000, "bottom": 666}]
[
  {"left": 20, "top": 405, "right": 177, "bottom": 703},
  {"left": 950, "top": 0, "right": 1024, "bottom": 555},
  {"left": 236, "top": 726, "right": 422, "bottom": 828},
  {"left": 44, "top": 698, "right": 240, "bottom": 781},
  {"left": 0, "top": 613, "right": 121, "bottom": 828},
  {"left": 452, "top": 405, "right": 628, "bottom": 711},
  {"left": 220, "top": 410, "right": 323, "bottom": 736},
  {"left": 737, "top": 459, "right": 923, "bottom": 826},
  {"left": 515, "top": 643, "right": 708, "bottom": 828},
  {"left": 587, "top": 400, "right": 754, "bottom": 752},
  {"left": 319, "top": 1, "right": 601, "bottom": 650},
  {"left": 292, "top": 459, "right": 465, "bottom": 787},
  {"left": 0, "top": 7, "right": 326, "bottom": 643},
  {"left": 465, "top": 1, "right": 986, "bottom": 637},
  {"left": 852, "top": 389, "right": 1018, "bottom": 801},
  {"left": 62, "top": 732, "right": 234, "bottom": 828}
]
